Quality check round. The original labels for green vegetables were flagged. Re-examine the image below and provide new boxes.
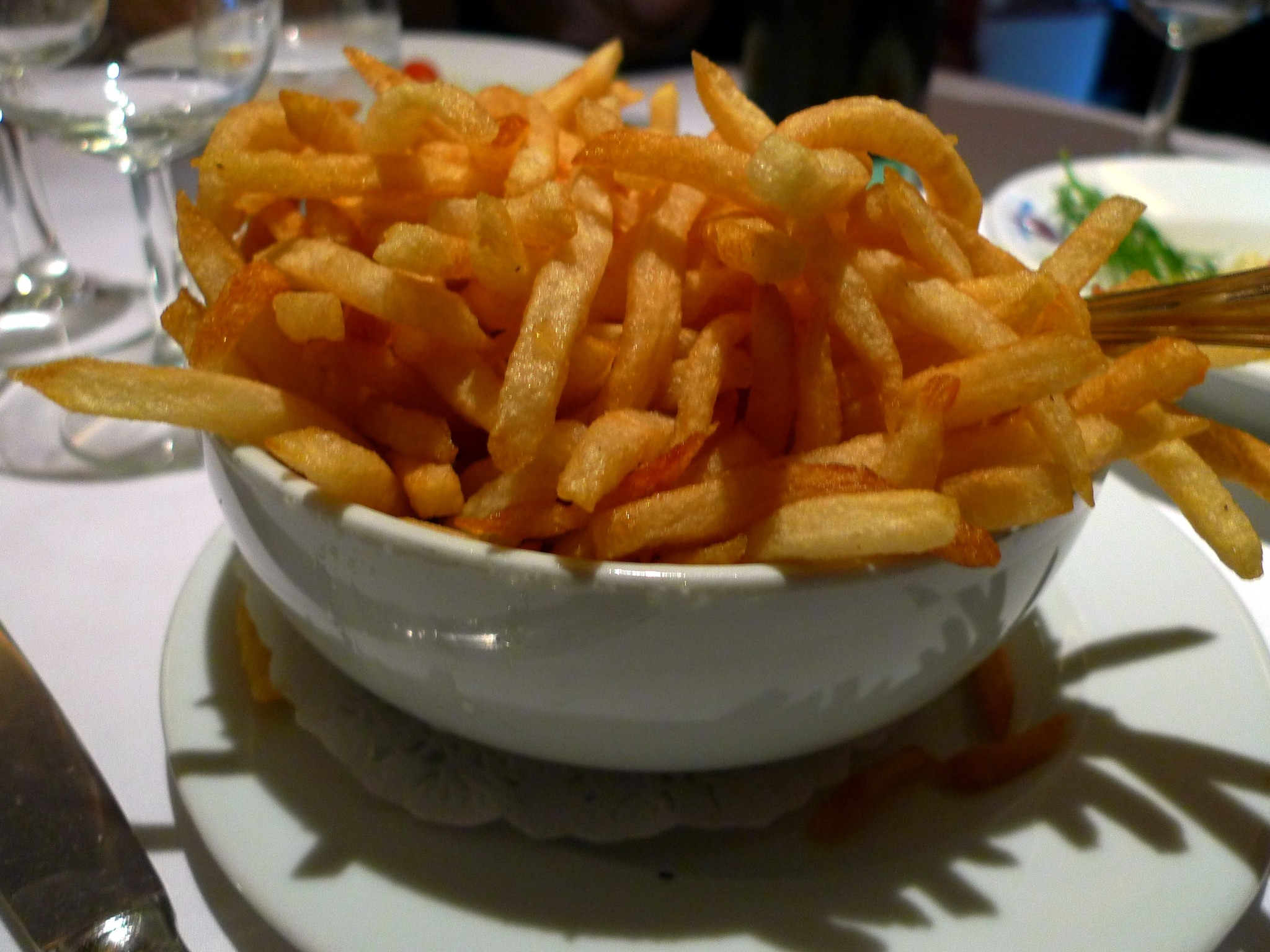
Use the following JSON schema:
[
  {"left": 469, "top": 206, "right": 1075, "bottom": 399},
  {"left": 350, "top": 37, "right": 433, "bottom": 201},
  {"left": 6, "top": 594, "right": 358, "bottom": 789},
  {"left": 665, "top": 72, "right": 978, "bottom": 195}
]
[{"left": 1057, "top": 141, "right": 1216, "bottom": 286}]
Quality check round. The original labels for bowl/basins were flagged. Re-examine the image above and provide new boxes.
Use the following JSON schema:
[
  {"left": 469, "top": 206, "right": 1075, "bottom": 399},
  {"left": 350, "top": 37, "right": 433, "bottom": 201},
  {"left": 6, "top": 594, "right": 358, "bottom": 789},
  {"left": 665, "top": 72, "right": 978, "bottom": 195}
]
[
  {"left": 982, "top": 155, "right": 1270, "bottom": 437},
  {"left": 193, "top": 431, "right": 1096, "bottom": 777}
]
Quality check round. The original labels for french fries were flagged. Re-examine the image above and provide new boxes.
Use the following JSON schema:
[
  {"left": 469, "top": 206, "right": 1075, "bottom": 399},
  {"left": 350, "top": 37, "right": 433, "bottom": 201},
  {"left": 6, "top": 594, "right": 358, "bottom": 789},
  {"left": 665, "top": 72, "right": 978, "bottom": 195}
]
[{"left": 8, "top": 38, "right": 1270, "bottom": 585}]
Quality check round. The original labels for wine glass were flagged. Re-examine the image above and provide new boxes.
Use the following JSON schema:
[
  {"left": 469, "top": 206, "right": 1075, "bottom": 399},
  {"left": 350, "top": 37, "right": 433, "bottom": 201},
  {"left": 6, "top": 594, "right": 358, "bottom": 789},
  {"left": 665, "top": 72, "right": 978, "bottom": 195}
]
[
  {"left": 0, "top": 0, "right": 155, "bottom": 374},
  {"left": 55, "top": 0, "right": 282, "bottom": 484}
]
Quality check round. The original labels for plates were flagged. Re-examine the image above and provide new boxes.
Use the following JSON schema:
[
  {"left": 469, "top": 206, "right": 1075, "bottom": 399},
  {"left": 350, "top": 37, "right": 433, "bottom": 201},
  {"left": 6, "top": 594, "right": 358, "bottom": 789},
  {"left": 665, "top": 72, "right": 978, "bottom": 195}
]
[
  {"left": 0, "top": 36, "right": 653, "bottom": 295},
  {"left": 159, "top": 466, "right": 1269, "bottom": 952}
]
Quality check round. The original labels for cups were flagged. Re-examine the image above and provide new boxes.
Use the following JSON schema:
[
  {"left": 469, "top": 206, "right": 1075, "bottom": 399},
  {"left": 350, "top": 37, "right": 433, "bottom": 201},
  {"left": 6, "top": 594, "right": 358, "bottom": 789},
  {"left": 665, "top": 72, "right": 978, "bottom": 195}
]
[{"left": 264, "top": 0, "right": 404, "bottom": 128}]
